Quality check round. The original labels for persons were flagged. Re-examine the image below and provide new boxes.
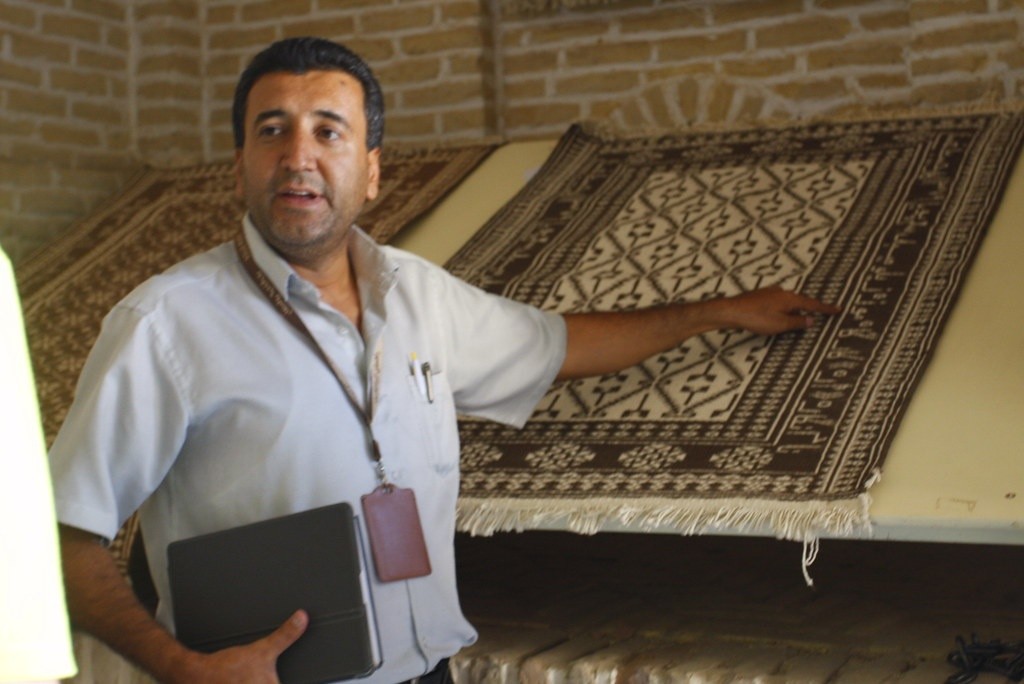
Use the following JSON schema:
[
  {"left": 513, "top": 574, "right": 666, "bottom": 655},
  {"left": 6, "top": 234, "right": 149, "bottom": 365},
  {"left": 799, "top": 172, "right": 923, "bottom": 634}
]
[
  {"left": 0, "top": 247, "right": 78, "bottom": 684},
  {"left": 48, "top": 37, "right": 840, "bottom": 684}
]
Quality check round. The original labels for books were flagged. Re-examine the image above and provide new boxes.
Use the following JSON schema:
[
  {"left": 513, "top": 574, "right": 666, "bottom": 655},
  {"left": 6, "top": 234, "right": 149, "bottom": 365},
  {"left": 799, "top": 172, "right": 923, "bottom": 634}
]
[{"left": 168, "top": 503, "right": 383, "bottom": 684}]
